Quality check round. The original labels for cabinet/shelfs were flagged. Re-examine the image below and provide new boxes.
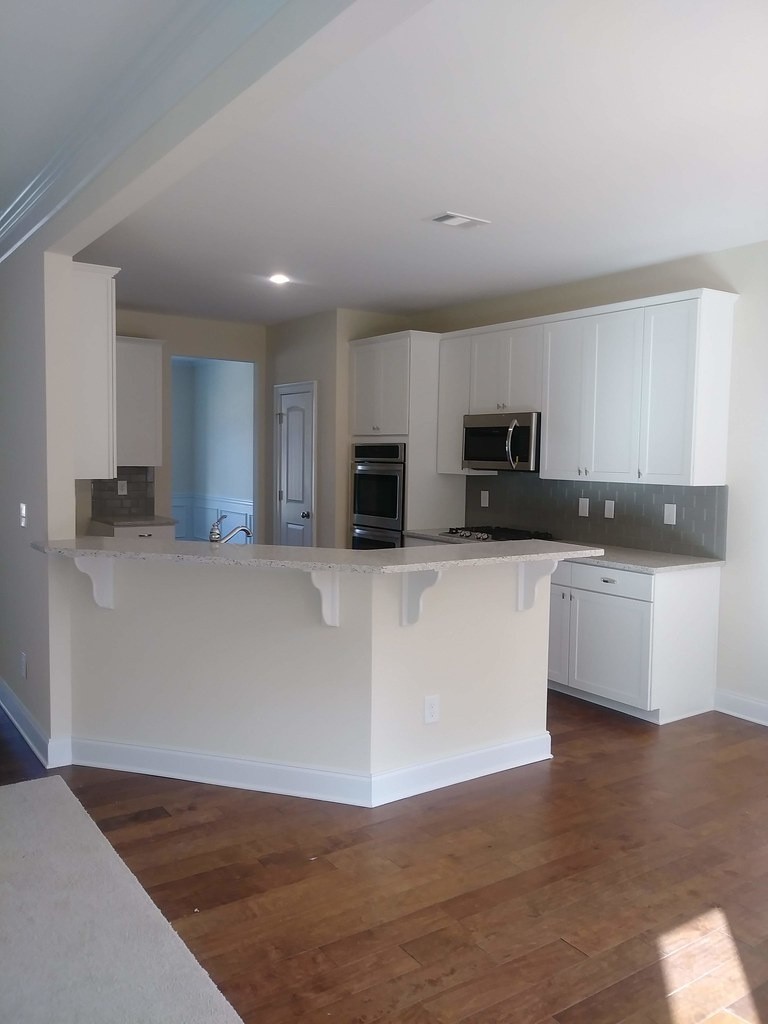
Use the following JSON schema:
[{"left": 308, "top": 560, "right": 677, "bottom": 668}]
[
  {"left": 435, "top": 334, "right": 471, "bottom": 475},
  {"left": 469, "top": 319, "right": 544, "bottom": 416},
  {"left": 116, "top": 334, "right": 164, "bottom": 469},
  {"left": 347, "top": 330, "right": 411, "bottom": 437},
  {"left": 548, "top": 559, "right": 660, "bottom": 726},
  {"left": 115, "top": 523, "right": 176, "bottom": 542},
  {"left": 538, "top": 300, "right": 645, "bottom": 483},
  {"left": 635, "top": 286, "right": 702, "bottom": 486}
]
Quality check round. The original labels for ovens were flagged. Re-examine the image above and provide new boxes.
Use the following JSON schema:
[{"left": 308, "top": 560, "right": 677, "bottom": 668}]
[{"left": 349, "top": 442, "right": 405, "bottom": 550}]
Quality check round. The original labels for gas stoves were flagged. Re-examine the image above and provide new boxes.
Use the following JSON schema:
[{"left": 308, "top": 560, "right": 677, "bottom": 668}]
[{"left": 439, "top": 525, "right": 563, "bottom": 543}]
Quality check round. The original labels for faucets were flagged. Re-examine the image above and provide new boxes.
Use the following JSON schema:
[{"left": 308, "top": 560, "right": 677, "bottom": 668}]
[{"left": 209, "top": 514, "right": 253, "bottom": 545}]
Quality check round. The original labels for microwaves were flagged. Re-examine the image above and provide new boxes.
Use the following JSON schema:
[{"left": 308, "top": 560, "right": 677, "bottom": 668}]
[{"left": 462, "top": 412, "right": 541, "bottom": 473}]
